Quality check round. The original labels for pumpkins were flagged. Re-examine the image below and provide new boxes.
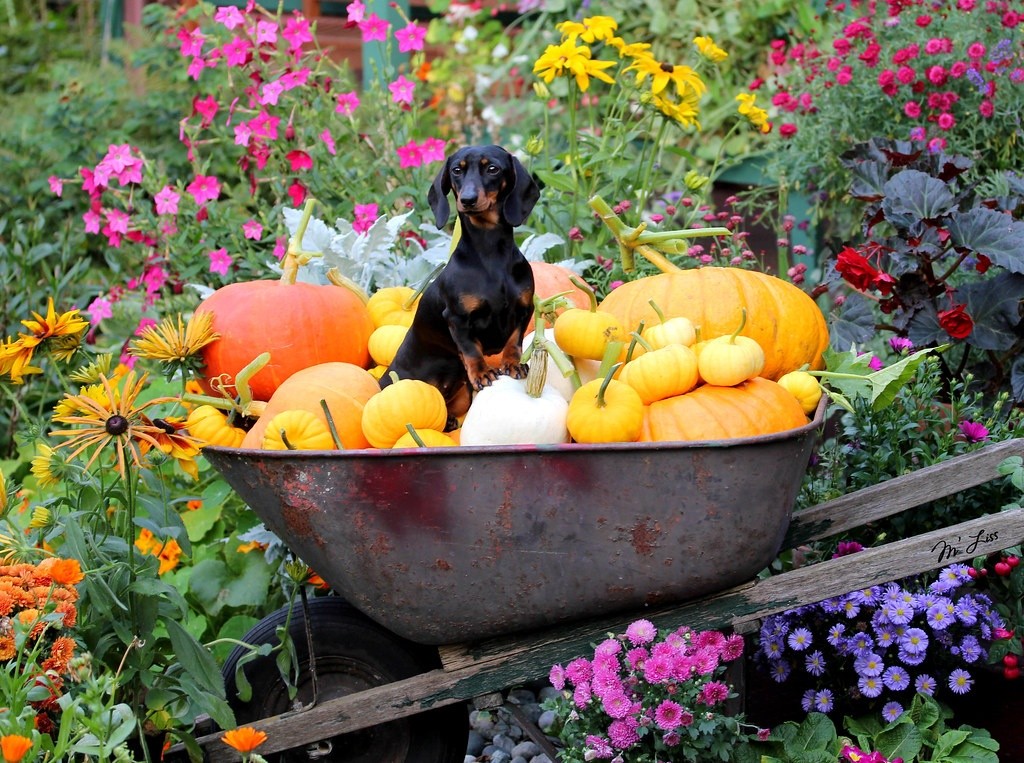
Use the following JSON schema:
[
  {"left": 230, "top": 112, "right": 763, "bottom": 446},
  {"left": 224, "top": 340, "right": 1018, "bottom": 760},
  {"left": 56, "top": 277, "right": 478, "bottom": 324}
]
[{"left": 185, "top": 259, "right": 829, "bottom": 450}]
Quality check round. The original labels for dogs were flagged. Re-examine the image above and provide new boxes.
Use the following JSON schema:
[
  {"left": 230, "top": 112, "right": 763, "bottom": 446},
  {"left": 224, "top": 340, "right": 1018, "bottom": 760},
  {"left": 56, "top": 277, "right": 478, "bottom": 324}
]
[{"left": 379, "top": 144, "right": 541, "bottom": 430}]
